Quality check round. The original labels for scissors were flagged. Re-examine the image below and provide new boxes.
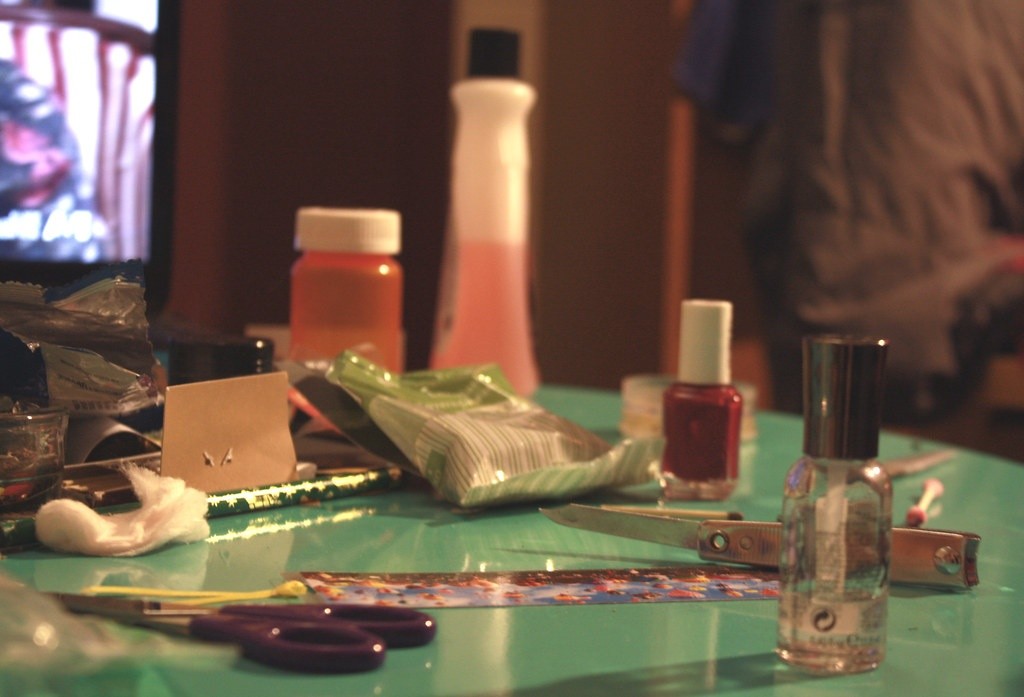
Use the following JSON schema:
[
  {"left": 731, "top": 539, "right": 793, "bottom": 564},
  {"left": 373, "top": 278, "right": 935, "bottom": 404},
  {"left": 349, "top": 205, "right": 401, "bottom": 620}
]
[{"left": 37, "top": 589, "right": 438, "bottom": 675}]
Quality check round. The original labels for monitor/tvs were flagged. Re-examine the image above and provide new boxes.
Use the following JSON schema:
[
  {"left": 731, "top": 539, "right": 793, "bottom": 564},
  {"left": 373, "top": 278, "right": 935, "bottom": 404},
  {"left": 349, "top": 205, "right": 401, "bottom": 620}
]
[{"left": 0, "top": 0, "right": 181, "bottom": 310}]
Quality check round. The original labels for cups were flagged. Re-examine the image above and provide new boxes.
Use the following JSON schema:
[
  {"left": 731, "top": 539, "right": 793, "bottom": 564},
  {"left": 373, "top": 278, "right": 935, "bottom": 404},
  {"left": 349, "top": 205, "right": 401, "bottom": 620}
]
[{"left": 0, "top": 412, "right": 68, "bottom": 551}]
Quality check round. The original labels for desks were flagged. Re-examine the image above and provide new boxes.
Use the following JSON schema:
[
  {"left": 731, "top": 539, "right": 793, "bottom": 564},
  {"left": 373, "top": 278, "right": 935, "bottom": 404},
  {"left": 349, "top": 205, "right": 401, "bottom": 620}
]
[{"left": 1, "top": 385, "right": 1024, "bottom": 697}]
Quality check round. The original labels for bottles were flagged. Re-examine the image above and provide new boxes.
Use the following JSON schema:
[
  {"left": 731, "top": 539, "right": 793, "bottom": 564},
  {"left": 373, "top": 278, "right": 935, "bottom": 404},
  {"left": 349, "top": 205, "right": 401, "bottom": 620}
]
[
  {"left": 288, "top": 204, "right": 408, "bottom": 383},
  {"left": 426, "top": 24, "right": 544, "bottom": 401}
]
